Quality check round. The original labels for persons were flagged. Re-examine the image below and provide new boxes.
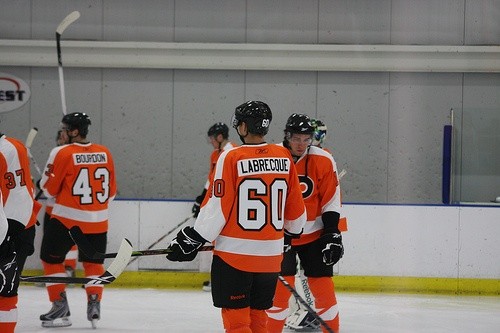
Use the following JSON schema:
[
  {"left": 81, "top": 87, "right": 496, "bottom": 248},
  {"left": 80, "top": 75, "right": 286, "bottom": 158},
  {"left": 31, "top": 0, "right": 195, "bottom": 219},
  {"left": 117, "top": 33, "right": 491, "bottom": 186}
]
[
  {"left": 0, "top": 112, "right": 117, "bottom": 333},
  {"left": 166, "top": 99, "right": 307, "bottom": 333},
  {"left": 265, "top": 113, "right": 344, "bottom": 333},
  {"left": 192, "top": 122, "right": 238, "bottom": 290}
]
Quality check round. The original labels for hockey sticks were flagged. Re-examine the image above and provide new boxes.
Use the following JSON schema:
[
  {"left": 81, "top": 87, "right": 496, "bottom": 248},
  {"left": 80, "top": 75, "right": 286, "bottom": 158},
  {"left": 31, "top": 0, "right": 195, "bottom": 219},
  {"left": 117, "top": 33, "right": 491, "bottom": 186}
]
[
  {"left": 338, "top": 168, "right": 347, "bottom": 180},
  {"left": 55, "top": 10, "right": 80, "bottom": 116},
  {"left": 26, "top": 127, "right": 43, "bottom": 178},
  {"left": 69, "top": 225, "right": 215, "bottom": 260},
  {"left": 277, "top": 274, "right": 335, "bottom": 333},
  {"left": 18, "top": 237, "right": 134, "bottom": 286},
  {"left": 127, "top": 212, "right": 195, "bottom": 265}
]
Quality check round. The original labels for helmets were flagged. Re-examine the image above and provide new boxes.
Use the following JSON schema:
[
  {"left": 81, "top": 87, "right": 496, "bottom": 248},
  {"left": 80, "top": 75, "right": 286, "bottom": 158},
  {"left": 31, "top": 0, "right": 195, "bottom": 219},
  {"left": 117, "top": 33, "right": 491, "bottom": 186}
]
[
  {"left": 285, "top": 113, "right": 314, "bottom": 135},
  {"left": 61, "top": 112, "right": 91, "bottom": 126},
  {"left": 207, "top": 122, "right": 229, "bottom": 139},
  {"left": 230, "top": 100, "right": 272, "bottom": 136},
  {"left": 311, "top": 118, "right": 327, "bottom": 141}
]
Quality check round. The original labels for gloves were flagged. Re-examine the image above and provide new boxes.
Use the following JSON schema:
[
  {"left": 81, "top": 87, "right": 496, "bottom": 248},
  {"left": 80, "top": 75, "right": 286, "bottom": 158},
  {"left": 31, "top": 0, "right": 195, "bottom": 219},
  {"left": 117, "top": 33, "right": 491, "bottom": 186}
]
[
  {"left": 192, "top": 195, "right": 205, "bottom": 218},
  {"left": 166, "top": 224, "right": 207, "bottom": 262},
  {"left": 321, "top": 231, "right": 344, "bottom": 266}
]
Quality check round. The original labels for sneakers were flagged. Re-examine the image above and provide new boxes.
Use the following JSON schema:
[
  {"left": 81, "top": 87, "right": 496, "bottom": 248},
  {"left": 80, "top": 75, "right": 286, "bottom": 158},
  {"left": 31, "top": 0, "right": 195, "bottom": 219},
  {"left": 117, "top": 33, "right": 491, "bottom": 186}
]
[
  {"left": 86, "top": 294, "right": 101, "bottom": 329},
  {"left": 286, "top": 308, "right": 321, "bottom": 333},
  {"left": 202, "top": 280, "right": 211, "bottom": 292},
  {"left": 39, "top": 299, "right": 72, "bottom": 327}
]
